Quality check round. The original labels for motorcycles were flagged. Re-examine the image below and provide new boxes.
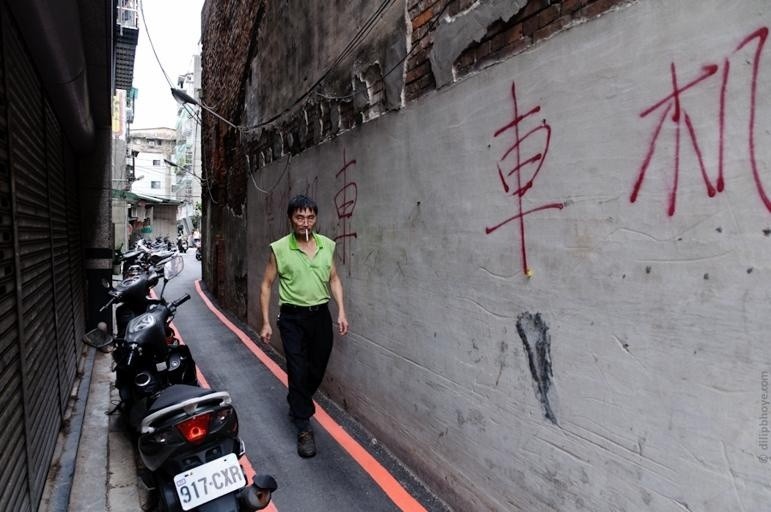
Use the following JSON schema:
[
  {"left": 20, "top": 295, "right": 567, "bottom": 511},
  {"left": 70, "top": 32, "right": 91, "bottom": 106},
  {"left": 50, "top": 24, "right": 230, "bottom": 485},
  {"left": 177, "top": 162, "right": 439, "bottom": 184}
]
[
  {"left": 115, "top": 229, "right": 202, "bottom": 280},
  {"left": 81, "top": 256, "right": 278, "bottom": 512},
  {"left": 97, "top": 249, "right": 185, "bottom": 363}
]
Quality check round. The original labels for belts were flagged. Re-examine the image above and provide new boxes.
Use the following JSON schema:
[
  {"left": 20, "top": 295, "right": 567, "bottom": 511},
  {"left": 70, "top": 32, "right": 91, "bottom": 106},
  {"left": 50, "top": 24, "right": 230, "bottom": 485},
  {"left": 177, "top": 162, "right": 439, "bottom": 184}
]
[{"left": 280, "top": 304, "right": 327, "bottom": 313}]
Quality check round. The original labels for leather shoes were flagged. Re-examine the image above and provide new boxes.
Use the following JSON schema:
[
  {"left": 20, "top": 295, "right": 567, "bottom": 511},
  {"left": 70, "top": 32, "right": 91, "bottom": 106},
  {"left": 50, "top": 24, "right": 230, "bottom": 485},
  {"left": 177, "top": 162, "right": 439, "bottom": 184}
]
[{"left": 298, "top": 430, "right": 316, "bottom": 458}]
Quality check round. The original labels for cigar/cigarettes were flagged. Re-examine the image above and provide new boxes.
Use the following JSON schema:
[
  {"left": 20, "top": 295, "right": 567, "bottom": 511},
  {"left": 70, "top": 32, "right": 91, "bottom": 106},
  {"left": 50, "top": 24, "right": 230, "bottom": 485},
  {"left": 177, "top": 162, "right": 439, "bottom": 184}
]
[{"left": 305, "top": 228, "right": 310, "bottom": 242}]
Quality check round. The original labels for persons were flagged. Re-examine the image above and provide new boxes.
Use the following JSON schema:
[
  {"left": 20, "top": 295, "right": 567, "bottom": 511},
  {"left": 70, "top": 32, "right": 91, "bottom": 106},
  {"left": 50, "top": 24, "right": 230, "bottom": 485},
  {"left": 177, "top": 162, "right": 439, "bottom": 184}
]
[
  {"left": 192, "top": 228, "right": 201, "bottom": 246},
  {"left": 260, "top": 193, "right": 350, "bottom": 460}
]
